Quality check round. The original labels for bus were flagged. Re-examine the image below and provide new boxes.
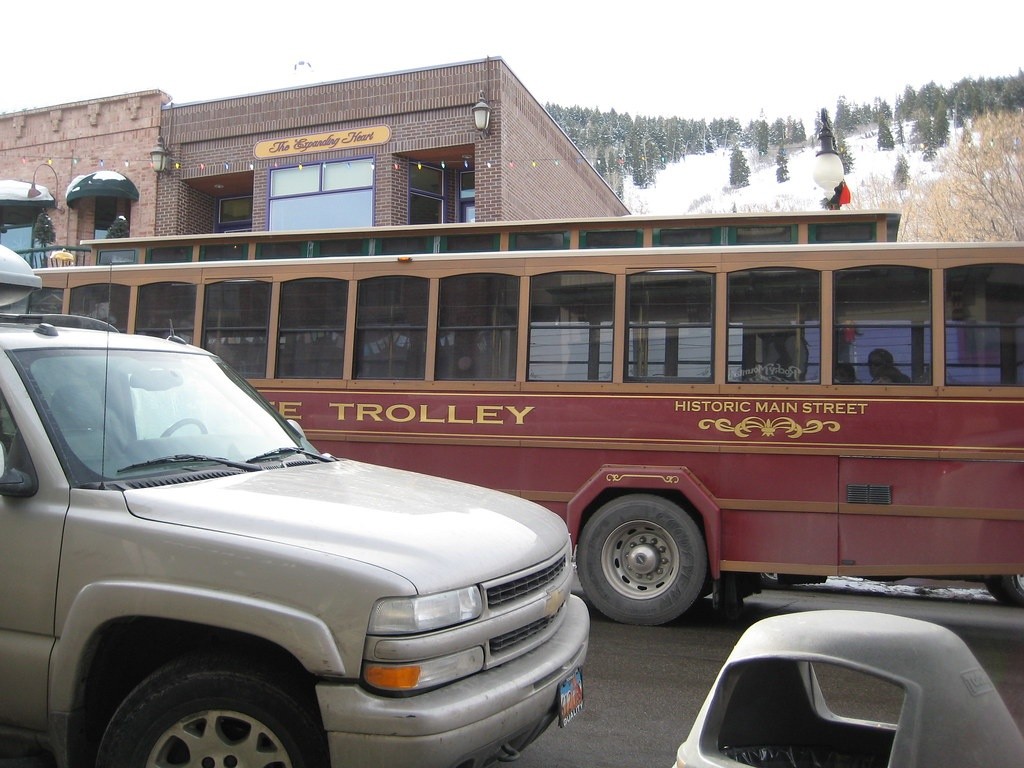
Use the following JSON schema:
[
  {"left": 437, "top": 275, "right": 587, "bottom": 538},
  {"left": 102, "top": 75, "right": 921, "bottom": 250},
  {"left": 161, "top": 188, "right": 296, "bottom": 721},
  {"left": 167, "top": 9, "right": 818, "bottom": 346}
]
[{"left": 39, "top": 240, "right": 1024, "bottom": 626}]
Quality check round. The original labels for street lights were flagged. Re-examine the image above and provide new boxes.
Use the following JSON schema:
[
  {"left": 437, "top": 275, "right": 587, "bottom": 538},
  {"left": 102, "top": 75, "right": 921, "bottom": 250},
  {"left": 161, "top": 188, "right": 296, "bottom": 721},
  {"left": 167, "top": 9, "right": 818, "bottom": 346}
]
[{"left": 813, "top": 108, "right": 844, "bottom": 212}]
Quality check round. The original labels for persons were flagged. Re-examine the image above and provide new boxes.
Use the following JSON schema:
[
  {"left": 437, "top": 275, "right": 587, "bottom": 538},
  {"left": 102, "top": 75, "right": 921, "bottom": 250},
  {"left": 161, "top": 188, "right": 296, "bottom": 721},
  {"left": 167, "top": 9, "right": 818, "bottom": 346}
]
[
  {"left": 747, "top": 331, "right": 809, "bottom": 382},
  {"left": 833, "top": 324, "right": 863, "bottom": 383},
  {"left": 867, "top": 348, "right": 911, "bottom": 384}
]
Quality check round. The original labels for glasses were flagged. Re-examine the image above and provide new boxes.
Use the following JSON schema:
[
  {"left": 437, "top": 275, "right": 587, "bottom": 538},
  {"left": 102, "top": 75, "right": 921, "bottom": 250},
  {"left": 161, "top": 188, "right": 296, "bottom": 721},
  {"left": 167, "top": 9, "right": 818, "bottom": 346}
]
[{"left": 867, "top": 358, "right": 886, "bottom": 366}]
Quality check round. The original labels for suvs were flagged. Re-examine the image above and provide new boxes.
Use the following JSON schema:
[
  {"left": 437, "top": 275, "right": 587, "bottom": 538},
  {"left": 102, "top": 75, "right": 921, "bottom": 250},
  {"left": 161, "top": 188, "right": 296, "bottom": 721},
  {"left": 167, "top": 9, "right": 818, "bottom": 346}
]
[{"left": 0, "top": 312, "right": 591, "bottom": 768}]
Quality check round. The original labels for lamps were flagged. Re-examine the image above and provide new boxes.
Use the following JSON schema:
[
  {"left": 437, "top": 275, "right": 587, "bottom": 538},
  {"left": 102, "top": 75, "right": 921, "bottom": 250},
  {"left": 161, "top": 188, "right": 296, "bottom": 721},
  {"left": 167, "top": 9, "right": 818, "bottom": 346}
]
[
  {"left": 472, "top": 89, "right": 493, "bottom": 137},
  {"left": 149, "top": 136, "right": 171, "bottom": 176},
  {"left": 27, "top": 163, "right": 65, "bottom": 215}
]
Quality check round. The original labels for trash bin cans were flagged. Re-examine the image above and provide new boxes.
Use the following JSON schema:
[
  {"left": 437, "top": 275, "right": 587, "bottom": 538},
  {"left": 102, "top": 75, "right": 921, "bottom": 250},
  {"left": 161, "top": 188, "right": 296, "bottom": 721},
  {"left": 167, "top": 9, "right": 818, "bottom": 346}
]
[{"left": 672, "top": 608, "right": 1024, "bottom": 768}]
